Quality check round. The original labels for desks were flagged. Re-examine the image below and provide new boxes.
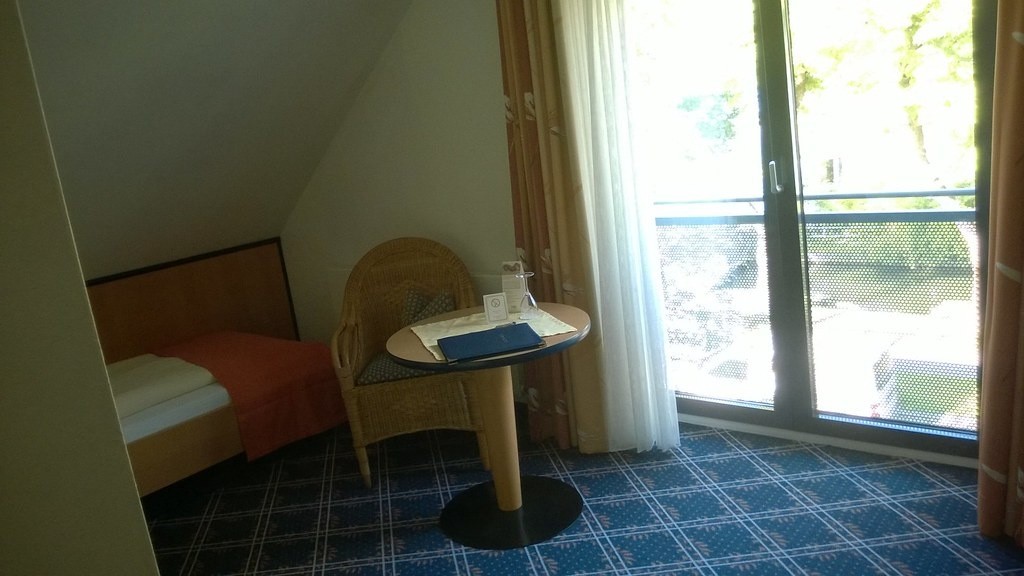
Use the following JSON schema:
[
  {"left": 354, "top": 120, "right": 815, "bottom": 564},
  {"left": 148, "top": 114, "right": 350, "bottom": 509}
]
[{"left": 385, "top": 302, "right": 590, "bottom": 550}]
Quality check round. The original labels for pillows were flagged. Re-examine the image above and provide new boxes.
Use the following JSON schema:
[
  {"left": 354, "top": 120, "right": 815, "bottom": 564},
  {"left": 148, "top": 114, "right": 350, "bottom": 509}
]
[{"left": 402, "top": 286, "right": 457, "bottom": 330}]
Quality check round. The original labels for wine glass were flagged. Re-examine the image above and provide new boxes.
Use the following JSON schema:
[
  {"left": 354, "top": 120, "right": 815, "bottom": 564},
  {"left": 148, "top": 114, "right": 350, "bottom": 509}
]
[{"left": 516, "top": 272, "right": 539, "bottom": 319}]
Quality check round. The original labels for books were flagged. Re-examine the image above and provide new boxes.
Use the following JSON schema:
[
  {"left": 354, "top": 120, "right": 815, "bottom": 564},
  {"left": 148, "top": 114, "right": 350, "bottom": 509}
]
[{"left": 437, "top": 323, "right": 545, "bottom": 367}]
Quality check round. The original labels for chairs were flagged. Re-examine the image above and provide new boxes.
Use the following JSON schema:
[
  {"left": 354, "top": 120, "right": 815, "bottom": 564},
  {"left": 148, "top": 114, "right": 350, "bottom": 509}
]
[{"left": 330, "top": 235, "right": 490, "bottom": 490}]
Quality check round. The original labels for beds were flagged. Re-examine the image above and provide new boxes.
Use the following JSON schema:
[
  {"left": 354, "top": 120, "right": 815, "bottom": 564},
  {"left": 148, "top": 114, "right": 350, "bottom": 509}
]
[{"left": 108, "top": 328, "right": 347, "bottom": 498}]
